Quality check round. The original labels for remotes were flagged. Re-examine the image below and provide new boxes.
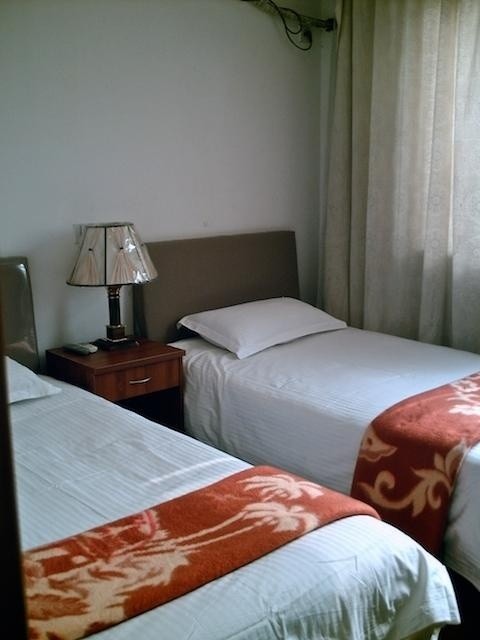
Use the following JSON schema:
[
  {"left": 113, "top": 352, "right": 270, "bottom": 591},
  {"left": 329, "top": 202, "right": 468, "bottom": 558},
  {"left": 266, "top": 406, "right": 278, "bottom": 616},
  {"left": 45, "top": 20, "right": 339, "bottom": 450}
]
[
  {"left": 77, "top": 341, "right": 99, "bottom": 354},
  {"left": 63, "top": 342, "right": 90, "bottom": 356}
]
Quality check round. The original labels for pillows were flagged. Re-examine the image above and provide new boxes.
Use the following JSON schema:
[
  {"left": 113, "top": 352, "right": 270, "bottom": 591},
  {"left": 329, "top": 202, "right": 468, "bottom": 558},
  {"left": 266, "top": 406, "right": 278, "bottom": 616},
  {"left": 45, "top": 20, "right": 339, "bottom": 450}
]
[
  {"left": 176, "top": 296, "right": 349, "bottom": 360},
  {"left": 4, "top": 355, "right": 62, "bottom": 406}
]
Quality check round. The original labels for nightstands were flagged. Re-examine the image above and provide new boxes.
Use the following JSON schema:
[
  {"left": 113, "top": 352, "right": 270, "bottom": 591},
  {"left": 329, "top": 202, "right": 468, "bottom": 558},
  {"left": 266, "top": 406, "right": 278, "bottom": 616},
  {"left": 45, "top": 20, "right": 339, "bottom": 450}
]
[{"left": 45, "top": 334, "right": 185, "bottom": 433}]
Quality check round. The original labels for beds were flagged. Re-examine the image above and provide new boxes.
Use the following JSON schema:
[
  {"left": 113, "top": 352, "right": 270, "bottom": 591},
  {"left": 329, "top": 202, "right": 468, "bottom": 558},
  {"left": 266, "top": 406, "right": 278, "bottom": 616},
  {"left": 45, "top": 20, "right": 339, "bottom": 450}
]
[
  {"left": 132, "top": 230, "right": 480, "bottom": 594},
  {"left": 0, "top": 256, "right": 462, "bottom": 640}
]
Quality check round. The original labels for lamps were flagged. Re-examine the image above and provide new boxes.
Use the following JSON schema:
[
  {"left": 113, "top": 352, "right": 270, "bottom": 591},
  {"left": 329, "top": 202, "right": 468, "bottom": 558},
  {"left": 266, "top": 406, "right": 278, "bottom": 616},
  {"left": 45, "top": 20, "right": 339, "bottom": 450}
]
[{"left": 66, "top": 222, "right": 159, "bottom": 352}]
